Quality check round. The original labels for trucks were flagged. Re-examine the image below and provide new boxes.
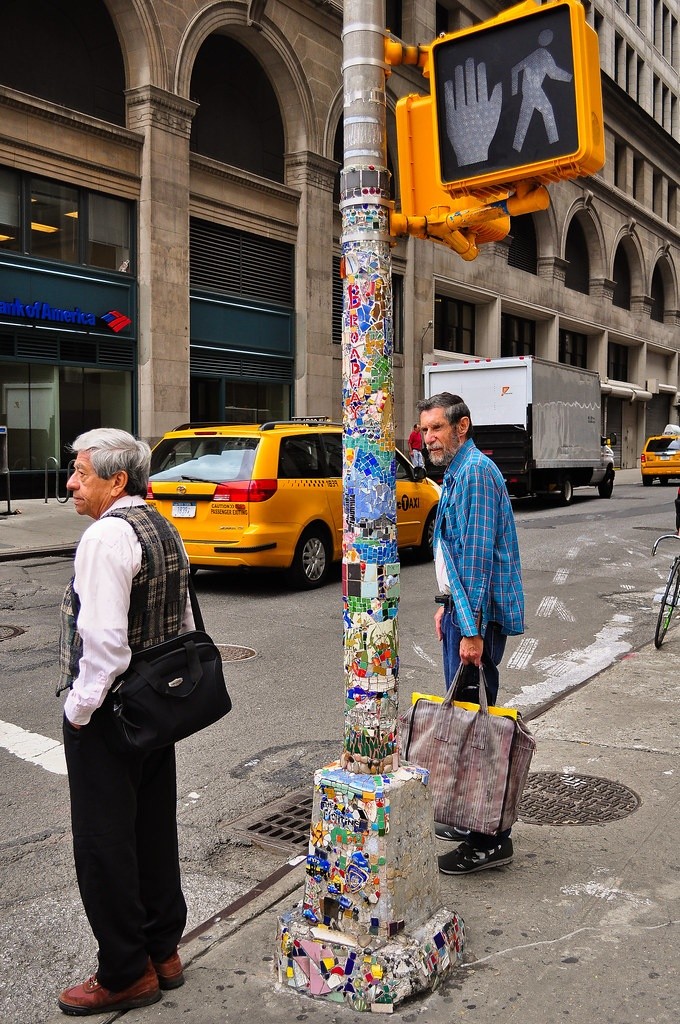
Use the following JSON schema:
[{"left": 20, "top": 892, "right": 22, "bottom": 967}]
[{"left": 421, "top": 352, "right": 617, "bottom": 505}]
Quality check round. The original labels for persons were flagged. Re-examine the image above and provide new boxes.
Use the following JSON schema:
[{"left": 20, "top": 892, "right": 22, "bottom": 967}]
[
  {"left": 409, "top": 424, "right": 425, "bottom": 468},
  {"left": 418, "top": 392, "right": 525, "bottom": 875},
  {"left": 673, "top": 486, "right": 680, "bottom": 536},
  {"left": 57, "top": 427, "right": 195, "bottom": 1017}
]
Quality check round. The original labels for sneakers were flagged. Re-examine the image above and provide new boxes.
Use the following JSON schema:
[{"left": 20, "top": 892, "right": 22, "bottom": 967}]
[
  {"left": 58, "top": 956, "right": 161, "bottom": 1014},
  {"left": 438, "top": 837, "right": 514, "bottom": 875},
  {"left": 435, "top": 825, "right": 473, "bottom": 840},
  {"left": 148, "top": 949, "right": 185, "bottom": 989}
]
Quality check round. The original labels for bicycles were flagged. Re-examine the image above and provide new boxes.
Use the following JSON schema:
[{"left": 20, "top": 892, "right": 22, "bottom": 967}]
[{"left": 650, "top": 535, "right": 680, "bottom": 649}]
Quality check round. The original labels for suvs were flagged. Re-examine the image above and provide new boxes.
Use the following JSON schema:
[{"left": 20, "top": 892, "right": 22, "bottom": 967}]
[
  {"left": 143, "top": 413, "right": 444, "bottom": 589},
  {"left": 639, "top": 435, "right": 680, "bottom": 486}
]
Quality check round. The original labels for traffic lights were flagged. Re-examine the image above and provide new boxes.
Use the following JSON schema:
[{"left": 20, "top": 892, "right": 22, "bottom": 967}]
[{"left": 426, "top": 0, "right": 608, "bottom": 200}]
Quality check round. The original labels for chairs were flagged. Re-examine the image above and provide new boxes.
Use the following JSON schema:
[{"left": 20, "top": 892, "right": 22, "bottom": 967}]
[
  {"left": 329, "top": 448, "right": 342, "bottom": 476},
  {"left": 283, "top": 447, "right": 310, "bottom": 472}
]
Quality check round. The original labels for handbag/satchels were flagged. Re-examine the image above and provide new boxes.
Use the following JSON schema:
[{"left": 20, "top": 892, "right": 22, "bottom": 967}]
[
  {"left": 394, "top": 660, "right": 537, "bottom": 836},
  {"left": 107, "top": 630, "right": 232, "bottom": 748}
]
